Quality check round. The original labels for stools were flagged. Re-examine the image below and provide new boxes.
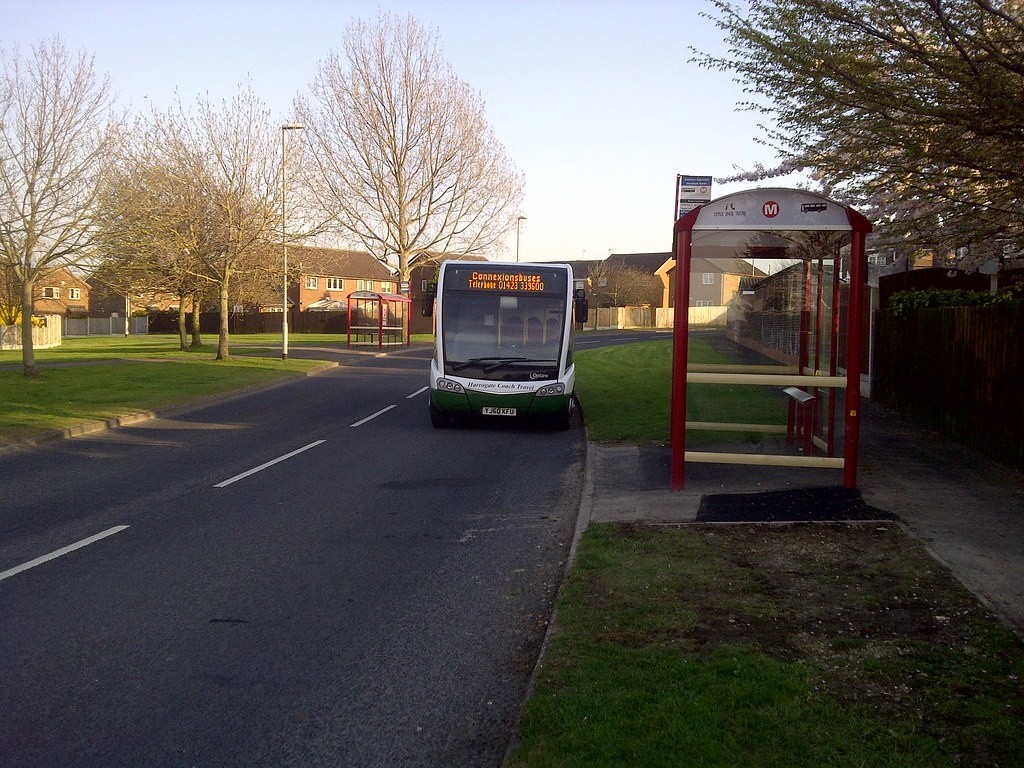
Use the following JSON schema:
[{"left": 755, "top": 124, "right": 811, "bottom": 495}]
[{"left": 783, "top": 386, "right": 816, "bottom": 455}]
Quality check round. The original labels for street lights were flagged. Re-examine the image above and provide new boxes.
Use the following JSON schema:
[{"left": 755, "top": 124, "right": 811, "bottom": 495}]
[
  {"left": 280, "top": 124, "right": 306, "bottom": 361},
  {"left": 515, "top": 216, "right": 526, "bottom": 264}
]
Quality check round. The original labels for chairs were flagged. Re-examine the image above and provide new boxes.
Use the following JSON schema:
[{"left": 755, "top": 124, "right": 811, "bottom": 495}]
[
  {"left": 546, "top": 317, "right": 560, "bottom": 344},
  {"left": 528, "top": 317, "right": 544, "bottom": 342},
  {"left": 503, "top": 317, "right": 523, "bottom": 337},
  {"left": 453, "top": 328, "right": 498, "bottom": 359}
]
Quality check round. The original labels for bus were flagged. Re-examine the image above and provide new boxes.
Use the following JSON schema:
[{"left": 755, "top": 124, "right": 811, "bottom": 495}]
[{"left": 422, "top": 260, "right": 590, "bottom": 429}]
